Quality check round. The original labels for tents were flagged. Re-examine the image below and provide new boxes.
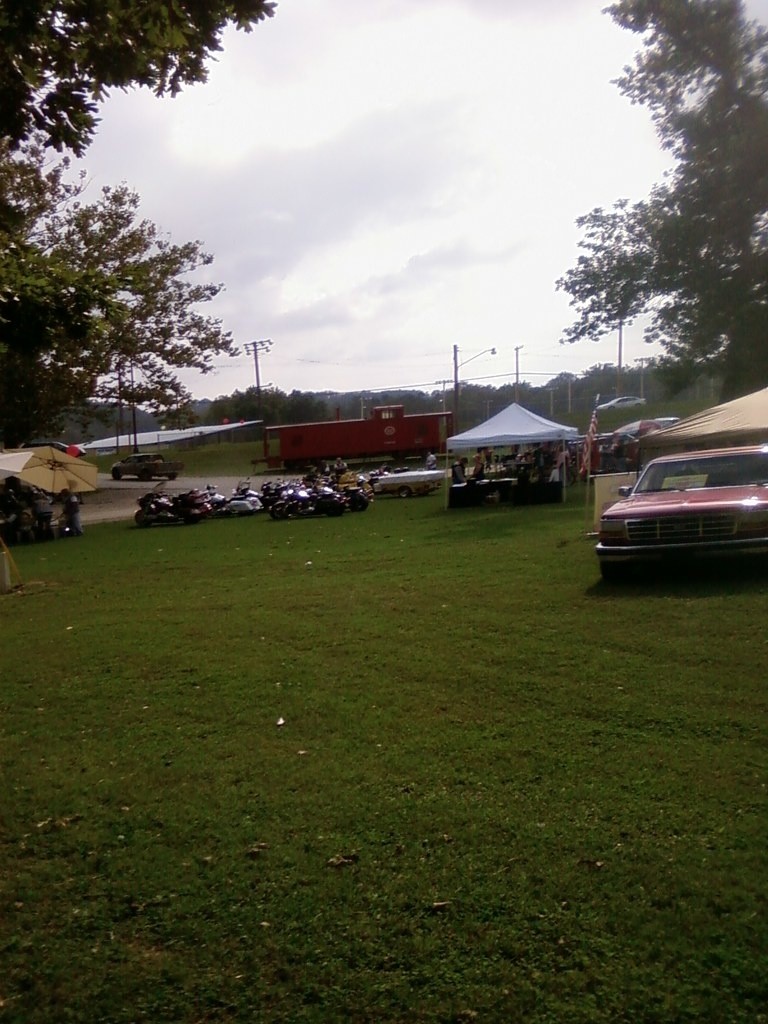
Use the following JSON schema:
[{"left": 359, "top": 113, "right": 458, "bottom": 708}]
[{"left": 445, "top": 402, "right": 580, "bottom": 511}]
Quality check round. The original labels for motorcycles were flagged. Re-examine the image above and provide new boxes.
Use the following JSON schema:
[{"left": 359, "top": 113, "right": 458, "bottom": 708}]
[
  {"left": 204, "top": 468, "right": 375, "bottom": 521},
  {"left": 135, "top": 479, "right": 212, "bottom": 527}
]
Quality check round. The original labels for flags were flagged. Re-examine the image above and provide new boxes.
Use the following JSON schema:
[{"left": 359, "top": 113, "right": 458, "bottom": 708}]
[{"left": 583, "top": 397, "right": 598, "bottom": 466}]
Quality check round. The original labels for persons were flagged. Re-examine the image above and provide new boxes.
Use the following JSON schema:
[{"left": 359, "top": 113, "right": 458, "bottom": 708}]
[
  {"left": 321, "top": 460, "right": 331, "bottom": 476},
  {"left": 452, "top": 447, "right": 493, "bottom": 484},
  {"left": 333, "top": 457, "right": 347, "bottom": 475},
  {"left": 533, "top": 442, "right": 546, "bottom": 480},
  {"left": 31, "top": 492, "right": 54, "bottom": 541},
  {"left": 61, "top": 488, "right": 83, "bottom": 537},
  {"left": 425, "top": 452, "right": 441, "bottom": 486},
  {"left": 613, "top": 439, "right": 625, "bottom": 472}
]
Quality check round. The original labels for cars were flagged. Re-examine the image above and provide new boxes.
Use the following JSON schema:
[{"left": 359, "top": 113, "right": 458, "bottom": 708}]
[
  {"left": 595, "top": 442, "right": 768, "bottom": 574},
  {"left": 595, "top": 396, "right": 647, "bottom": 410}
]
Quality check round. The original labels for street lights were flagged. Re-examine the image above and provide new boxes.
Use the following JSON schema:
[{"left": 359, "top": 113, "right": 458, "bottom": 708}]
[
  {"left": 244, "top": 339, "right": 275, "bottom": 423},
  {"left": 454, "top": 343, "right": 498, "bottom": 433}
]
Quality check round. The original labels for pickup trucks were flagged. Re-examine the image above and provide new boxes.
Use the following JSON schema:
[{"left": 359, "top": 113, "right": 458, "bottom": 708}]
[{"left": 112, "top": 452, "right": 184, "bottom": 481}]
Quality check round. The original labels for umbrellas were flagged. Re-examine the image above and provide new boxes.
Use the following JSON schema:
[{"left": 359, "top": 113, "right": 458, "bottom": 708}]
[
  {"left": 0, "top": 446, "right": 100, "bottom": 494},
  {"left": 614, "top": 418, "right": 658, "bottom": 435}
]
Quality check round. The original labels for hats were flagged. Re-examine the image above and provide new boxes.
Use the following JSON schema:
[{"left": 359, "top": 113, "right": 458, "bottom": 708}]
[
  {"left": 472, "top": 453, "right": 481, "bottom": 461},
  {"left": 461, "top": 458, "right": 468, "bottom": 464}
]
